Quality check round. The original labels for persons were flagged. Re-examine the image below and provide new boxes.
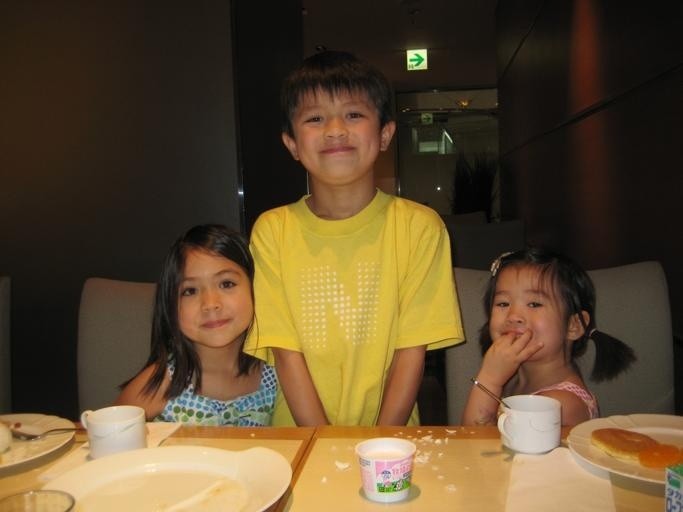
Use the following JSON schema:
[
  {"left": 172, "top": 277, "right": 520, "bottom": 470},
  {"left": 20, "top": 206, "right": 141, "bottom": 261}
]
[
  {"left": 101, "top": 221, "right": 279, "bottom": 426},
  {"left": 456, "top": 242, "right": 639, "bottom": 427},
  {"left": 238, "top": 49, "right": 469, "bottom": 429}
]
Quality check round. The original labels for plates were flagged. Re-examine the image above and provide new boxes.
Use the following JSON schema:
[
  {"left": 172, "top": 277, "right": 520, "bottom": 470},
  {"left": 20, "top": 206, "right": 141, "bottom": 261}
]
[
  {"left": 0, "top": 412, "right": 74, "bottom": 471},
  {"left": 565, "top": 410, "right": 682, "bottom": 483},
  {"left": 41, "top": 444, "right": 293, "bottom": 512}
]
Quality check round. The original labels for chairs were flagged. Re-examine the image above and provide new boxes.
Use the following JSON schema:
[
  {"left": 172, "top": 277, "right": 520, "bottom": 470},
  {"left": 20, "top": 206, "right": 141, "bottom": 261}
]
[
  {"left": 74, "top": 277, "right": 187, "bottom": 426},
  {"left": 0, "top": 274, "right": 11, "bottom": 416},
  {"left": 447, "top": 261, "right": 677, "bottom": 434}
]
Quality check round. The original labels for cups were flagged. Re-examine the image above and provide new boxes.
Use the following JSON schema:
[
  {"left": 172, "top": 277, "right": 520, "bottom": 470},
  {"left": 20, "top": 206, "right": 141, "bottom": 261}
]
[
  {"left": 80, "top": 405, "right": 145, "bottom": 456},
  {"left": 354, "top": 438, "right": 416, "bottom": 502},
  {"left": 498, "top": 392, "right": 563, "bottom": 455},
  {"left": 0, "top": 489, "right": 74, "bottom": 512}
]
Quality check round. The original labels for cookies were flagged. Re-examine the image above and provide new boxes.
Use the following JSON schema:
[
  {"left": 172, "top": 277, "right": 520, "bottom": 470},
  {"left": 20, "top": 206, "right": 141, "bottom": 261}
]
[
  {"left": 590, "top": 428, "right": 658, "bottom": 463},
  {"left": 640, "top": 443, "right": 682, "bottom": 468}
]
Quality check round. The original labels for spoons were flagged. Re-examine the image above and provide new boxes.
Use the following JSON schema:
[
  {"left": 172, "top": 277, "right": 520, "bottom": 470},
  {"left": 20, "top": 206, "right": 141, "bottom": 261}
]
[{"left": 10, "top": 426, "right": 85, "bottom": 443}]
